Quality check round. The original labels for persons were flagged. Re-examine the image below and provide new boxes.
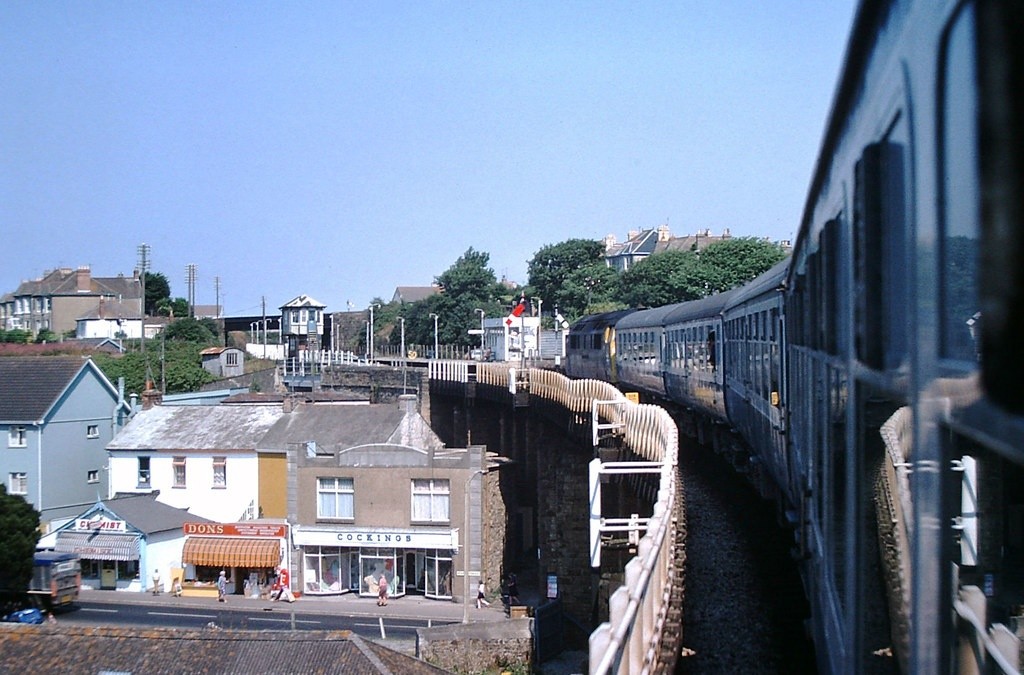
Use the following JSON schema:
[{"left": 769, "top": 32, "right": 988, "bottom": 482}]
[
  {"left": 152, "top": 569, "right": 160, "bottom": 596},
  {"left": 508, "top": 573, "right": 521, "bottom": 605},
  {"left": 171, "top": 576, "right": 182, "bottom": 597},
  {"left": 477, "top": 580, "right": 490, "bottom": 609},
  {"left": 378, "top": 574, "right": 388, "bottom": 606},
  {"left": 217, "top": 570, "right": 230, "bottom": 602}
]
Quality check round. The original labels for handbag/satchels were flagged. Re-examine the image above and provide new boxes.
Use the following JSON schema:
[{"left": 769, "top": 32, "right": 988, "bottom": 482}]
[{"left": 478, "top": 593, "right": 484, "bottom": 598}]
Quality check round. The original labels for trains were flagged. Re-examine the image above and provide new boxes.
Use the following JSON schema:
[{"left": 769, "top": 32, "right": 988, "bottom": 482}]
[{"left": 565, "top": 1, "right": 1024, "bottom": 675}]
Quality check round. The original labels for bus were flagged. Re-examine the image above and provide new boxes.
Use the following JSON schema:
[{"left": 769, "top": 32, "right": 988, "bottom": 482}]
[{"left": 28, "top": 551, "right": 81, "bottom": 609}]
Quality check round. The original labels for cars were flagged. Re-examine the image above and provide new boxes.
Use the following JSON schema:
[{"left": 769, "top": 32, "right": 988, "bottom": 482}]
[
  {"left": 4, "top": 607, "right": 44, "bottom": 624},
  {"left": 465, "top": 349, "right": 486, "bottom": 362}
]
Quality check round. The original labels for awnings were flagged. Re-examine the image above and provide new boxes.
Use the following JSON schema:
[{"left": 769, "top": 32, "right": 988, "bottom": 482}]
[
  {"left": 181, "top": 537, "right": 281, "bottom": 568},
  {"left": 54, "top": 533, "right": 140, "bottom": 561},
  {"left": 292, "top": 525, "right": 463, "bottom": 555}
]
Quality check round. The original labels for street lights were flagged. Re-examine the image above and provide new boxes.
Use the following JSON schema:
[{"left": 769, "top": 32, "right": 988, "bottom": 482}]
[
  {"left": 329, "top": 314, "right": 334, "bottom": 354},
  {"left": 370, "top": 303, "right": 381, "bottom": 363},
  {"left": 396, "top": 316, "right": 404, "bottom": 358},
  {"left": 474, "top": 308, "right": 484, "bottom": 349},
  {"left": 337, "top": 324, "right": 340, "bottom": 352},
  {"left": 361, "top": 319, "right": 369, "bottom": 355},
  {"left": 429, "top": 313, "right": 439, "bottom": 359},
  {"left": 537, "top": 299, "right": 542, "bottom": 357},
  {"left": 250, "top": 318, "right": 272, "bottom": 344}
]
